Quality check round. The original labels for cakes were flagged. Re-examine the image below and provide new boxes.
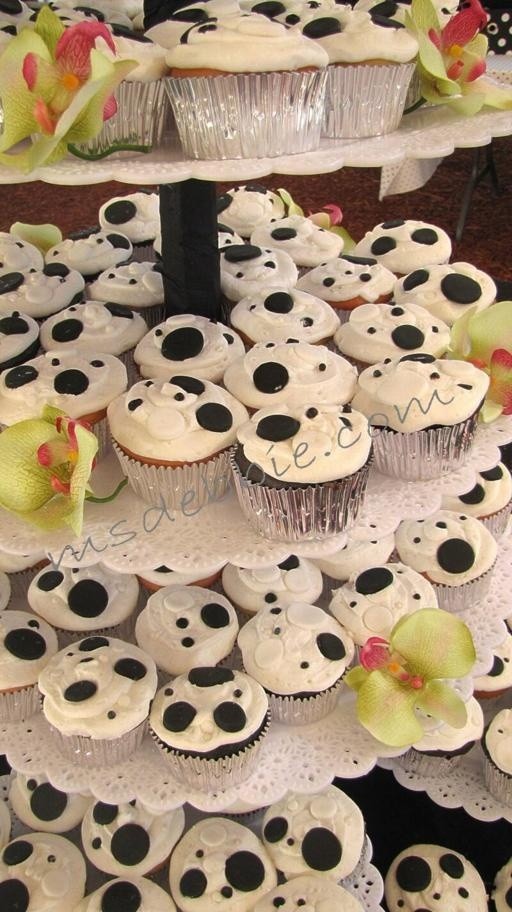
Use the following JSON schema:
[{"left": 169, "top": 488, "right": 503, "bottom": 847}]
[{"left": 0, "top": 0, "right": 452, "bottom": 445}]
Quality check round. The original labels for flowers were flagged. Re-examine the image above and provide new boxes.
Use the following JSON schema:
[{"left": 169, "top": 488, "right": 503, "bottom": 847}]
[
  {"left": 442, "top": 301, "right": 512, "bottom": 423},
  {"left": 343, "top": 606, "right": 476, "bottom": 747},
  {"left": 403, "top": 0, "right": 512, "bottom": 117},
  {"left": 276, "top": 187, "right": 357, "bottom": 258},
  {"left": 0, "top": 5, "right": 153, "bottom": 176},
  {"left": 0, "top": 403, "right": 129, "bottom": 539}
]
